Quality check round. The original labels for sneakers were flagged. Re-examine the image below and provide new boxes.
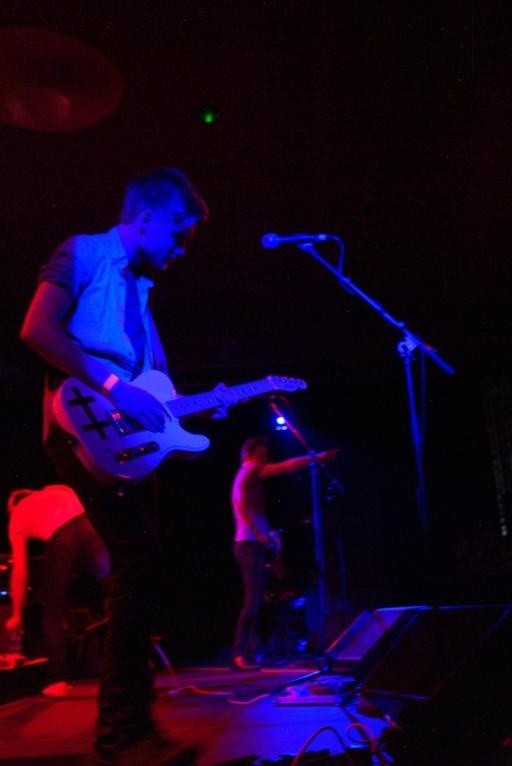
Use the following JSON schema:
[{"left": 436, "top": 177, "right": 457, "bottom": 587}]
[
  {"left": 42, "top": 680, "right": 72, "bottom": 698},
  {"left": 95, "top": 724, "right": 202, "bottom": 763}
]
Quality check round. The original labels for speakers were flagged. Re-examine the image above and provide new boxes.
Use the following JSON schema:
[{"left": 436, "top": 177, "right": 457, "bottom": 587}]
[{"left": 324, "top": 601, "right": 512, "bottom": 707}]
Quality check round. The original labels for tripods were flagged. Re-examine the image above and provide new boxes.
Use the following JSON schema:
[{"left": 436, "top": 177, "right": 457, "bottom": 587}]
[{"left": 255, "top": 407, "right": 347, "bottom": 695}]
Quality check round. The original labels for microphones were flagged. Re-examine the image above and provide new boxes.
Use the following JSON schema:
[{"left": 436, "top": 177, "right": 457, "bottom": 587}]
[{"left": 259, "top": 232, "right": 331, "bottom": 250}]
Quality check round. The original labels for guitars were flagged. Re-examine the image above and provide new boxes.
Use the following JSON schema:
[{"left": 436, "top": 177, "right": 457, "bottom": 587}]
[{"left": 51, "top": 370, "right": 308, "bottom": 483}]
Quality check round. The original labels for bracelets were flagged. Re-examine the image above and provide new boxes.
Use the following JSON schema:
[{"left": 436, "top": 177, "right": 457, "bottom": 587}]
[{"left": 102, "top": 371, "right": 121, "bottom": 394}]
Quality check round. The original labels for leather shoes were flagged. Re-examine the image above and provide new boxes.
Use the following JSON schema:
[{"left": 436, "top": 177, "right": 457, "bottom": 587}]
[{"left": 231, "top": 656, "right": 261, "bottom": 672}]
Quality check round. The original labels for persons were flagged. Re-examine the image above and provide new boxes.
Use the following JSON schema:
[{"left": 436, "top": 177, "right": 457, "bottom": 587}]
[
  {"left": 1, "top": 479, "right": 197, "bottom": 697},
  {"left": 19, "top": 153, "right": 236, "bottom": 764},
  {"left": 230, "top": 435, "right": 343, "bottom": 674}
]
